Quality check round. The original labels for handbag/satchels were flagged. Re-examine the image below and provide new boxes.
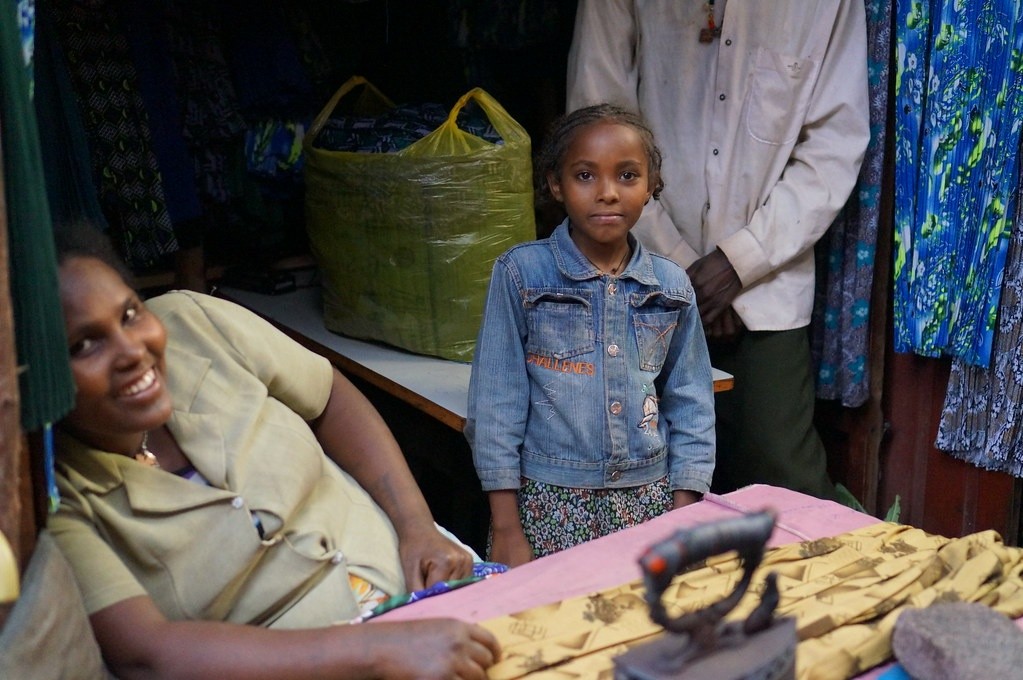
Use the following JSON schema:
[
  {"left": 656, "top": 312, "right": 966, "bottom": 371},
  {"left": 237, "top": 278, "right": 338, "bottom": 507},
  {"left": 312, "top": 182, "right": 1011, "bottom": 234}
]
[{"left": 306, "top": 76, "right": 536, "bottom": 366}]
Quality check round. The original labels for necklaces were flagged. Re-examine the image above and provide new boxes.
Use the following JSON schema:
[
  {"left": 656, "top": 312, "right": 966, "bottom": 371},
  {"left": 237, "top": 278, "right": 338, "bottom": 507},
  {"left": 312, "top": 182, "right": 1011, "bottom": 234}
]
[
  {"left": 572, "top": 236, "right": 628, "bottom": 274},
  {"left": 134, "top": 432, "right": 161, "bottom": 466}
]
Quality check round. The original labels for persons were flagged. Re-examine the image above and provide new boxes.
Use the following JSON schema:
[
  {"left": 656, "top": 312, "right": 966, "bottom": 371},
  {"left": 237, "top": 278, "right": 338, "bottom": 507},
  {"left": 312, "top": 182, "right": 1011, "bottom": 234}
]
[
  {"left": 464, "top": 103, "right": 717, "bottom": 570},
  {"left": 565, "top": 0, "right": 871, "bottom": 507},
  {"left": 41, "top": 252, "right": 501, "bottom": 680}
]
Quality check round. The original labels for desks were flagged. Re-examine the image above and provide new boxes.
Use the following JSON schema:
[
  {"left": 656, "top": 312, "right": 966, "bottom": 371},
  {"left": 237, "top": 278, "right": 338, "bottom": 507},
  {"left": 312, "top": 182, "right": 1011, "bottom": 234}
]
[
  {"left": 216, "top": 276, "right": 734, "bottom": 433},
  {"left": 369, "top": 481, "right": 1023, "bottom": 680}
]
